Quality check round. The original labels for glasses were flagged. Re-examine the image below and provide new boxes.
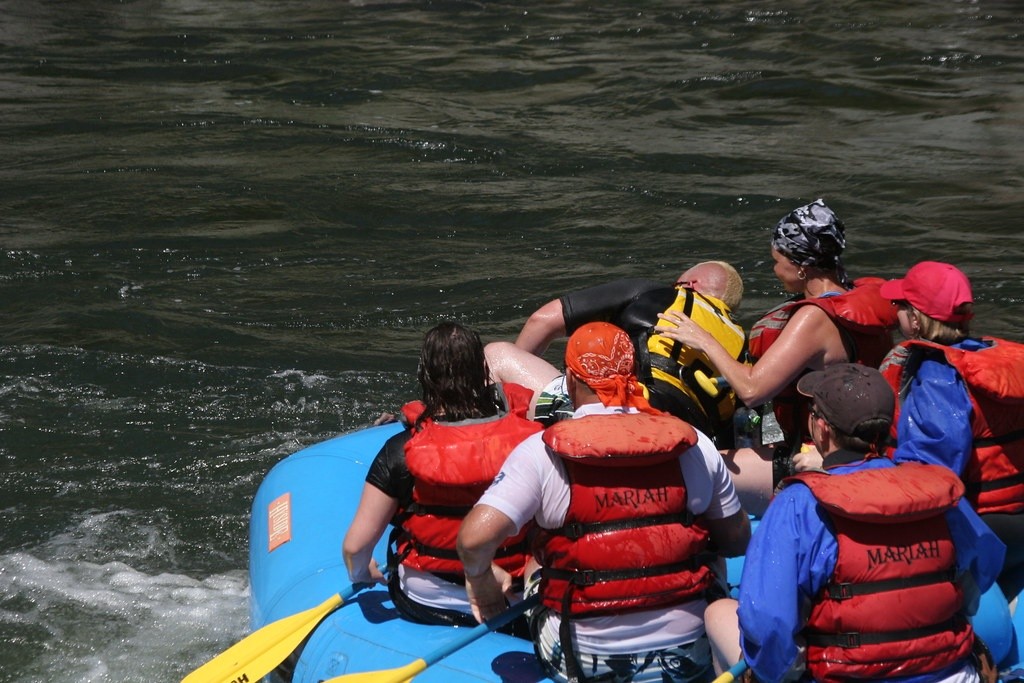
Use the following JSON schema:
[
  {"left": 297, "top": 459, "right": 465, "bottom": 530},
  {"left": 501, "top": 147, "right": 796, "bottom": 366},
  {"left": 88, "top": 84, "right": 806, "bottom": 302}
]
[{"left": 672, "top": 280, "right": 698, "bottom": 286}]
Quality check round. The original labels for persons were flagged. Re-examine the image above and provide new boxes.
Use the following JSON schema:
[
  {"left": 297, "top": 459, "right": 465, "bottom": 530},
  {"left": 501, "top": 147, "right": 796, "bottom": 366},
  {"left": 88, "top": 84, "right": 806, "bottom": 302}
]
[
  {"left": 457, "top": 323, "right": 751, "bottom": 683},
  {"left": 342, "top": 198, "right": 1024, "bottom": 628},
  {"left": 705, "top": 364, "right": 1008, "bottom": 683}
]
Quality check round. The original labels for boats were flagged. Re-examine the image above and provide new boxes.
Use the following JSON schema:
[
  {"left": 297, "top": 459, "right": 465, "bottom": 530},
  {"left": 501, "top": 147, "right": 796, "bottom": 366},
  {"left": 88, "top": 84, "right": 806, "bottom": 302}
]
[{"left": 249, "top": 421, "right": 760, "bottom": 683}]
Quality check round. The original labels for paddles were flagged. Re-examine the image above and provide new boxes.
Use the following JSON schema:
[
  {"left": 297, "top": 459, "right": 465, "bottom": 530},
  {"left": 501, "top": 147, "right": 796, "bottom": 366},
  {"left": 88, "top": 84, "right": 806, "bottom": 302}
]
[
  {"left": 317, "top": 594, "right": 532, "bottom": 683},
  {"left": 179, "top": 553, "right": 387, "bottom": 683}
]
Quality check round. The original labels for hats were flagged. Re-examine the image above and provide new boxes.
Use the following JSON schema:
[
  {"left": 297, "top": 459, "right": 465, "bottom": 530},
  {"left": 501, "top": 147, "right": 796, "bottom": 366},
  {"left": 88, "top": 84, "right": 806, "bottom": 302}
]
[
  {"left": 879, "top": 260, "right": 978, "bottom": 324},
  {"left": 798, "top": 361, "right": 899, "bottom": 443}
]
